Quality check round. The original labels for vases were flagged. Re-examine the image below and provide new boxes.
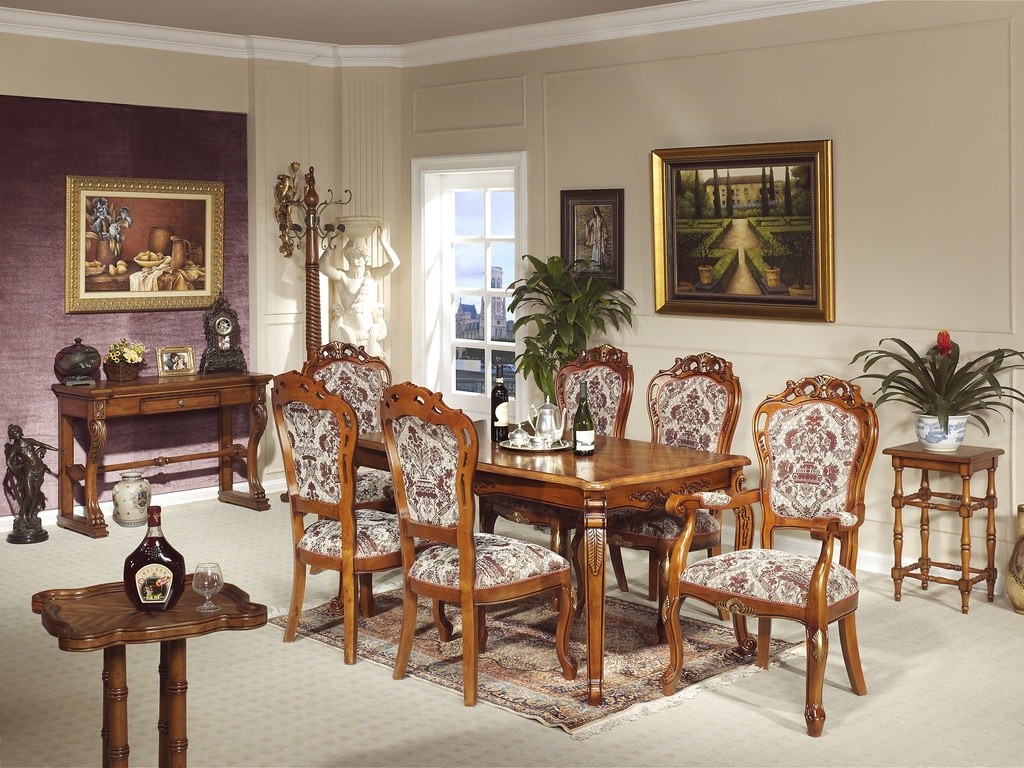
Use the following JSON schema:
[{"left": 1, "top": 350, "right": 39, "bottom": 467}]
[
  {"left": 1004, "top": 504, "right": 1024, "bottom": 615},
  {"left": 111, "top": 472, "right": 152, "bottom": 527},
  {"left": 914, "top": 413, "right": 971, "bottom": 452},
  {"left": 103, "top": 357, "right": 146, "bottom": 381}
]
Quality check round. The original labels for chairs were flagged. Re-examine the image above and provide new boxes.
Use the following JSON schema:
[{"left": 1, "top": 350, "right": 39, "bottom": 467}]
[
  {"left": 378, "top": 382, "right": 579, "bottom": 707},
  {"left": 658, "top": 375, "right": 879, "bottom": 738},
  {"left": 569, "top": 351, "right": 742, "bottom": 643},
  {"left": 301, "top": 340, "right": 398, "bottom": 615},
  {"left": 479, "top": 344, "right": 634, "bottom": 592},
  {"left": 269, "top": 369, "right": 455, "bottom": 668}
]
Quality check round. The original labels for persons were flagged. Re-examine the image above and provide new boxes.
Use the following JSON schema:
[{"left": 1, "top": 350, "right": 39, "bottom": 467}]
[
  {"left": 165, "top": 354, "right": 186, "bottom": 369},
  {"left": 318, "top": 223, "right": 401, "bottom": 356},
  {"left": 7, "top": 424, "right": 65, "bottom": 519}
]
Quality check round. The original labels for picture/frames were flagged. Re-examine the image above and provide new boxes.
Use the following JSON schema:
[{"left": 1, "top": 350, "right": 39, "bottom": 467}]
[
  {"left": 154, "top": 346, "right": 195, "bottom": 377},
  {"left": 560, "top": 187, "right": 625, "bottom": 289},
  {"left": 651, "top": 139, "right": 838, "bottom": 324},
  {"left": 65, "top": 174, "right": 224, "bottom": 314}
]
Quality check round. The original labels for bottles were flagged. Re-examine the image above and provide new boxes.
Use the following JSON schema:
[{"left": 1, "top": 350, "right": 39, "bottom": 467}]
[
  {"left": 573, "top": 380, "right": 594, "bottom": 456},
  {"left": 124, "top": 506, "right": 185, "bottom": 611},
  {"left": 491, "top": 365, "right": 509, "bottom": 443}
]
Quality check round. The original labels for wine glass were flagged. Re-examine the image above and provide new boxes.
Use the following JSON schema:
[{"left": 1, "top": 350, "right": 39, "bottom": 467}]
[{"left": 192, "top": 563, "right": 223, "bottom": 612}]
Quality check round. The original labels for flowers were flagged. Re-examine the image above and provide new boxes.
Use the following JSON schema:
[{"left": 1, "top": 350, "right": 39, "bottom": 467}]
[
  {"left": 102, "top": 337, "right": 151, "bottom": 365},
  {"left": 847, "top": 330, "right": 1024, "bottom": 436}
]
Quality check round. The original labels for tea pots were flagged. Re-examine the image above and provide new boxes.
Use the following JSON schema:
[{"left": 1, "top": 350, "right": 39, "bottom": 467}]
[{"left": 528, "top": 395, "right": 567, "bottom": 448}]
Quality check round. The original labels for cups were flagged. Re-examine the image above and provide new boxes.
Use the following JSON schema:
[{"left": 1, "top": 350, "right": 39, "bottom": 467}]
[
  {"left": 528, "top": 433, "right": 553, "bottom": 449},
  {"left": 508, "top": 422, "right": 531, "bottom": 449}
]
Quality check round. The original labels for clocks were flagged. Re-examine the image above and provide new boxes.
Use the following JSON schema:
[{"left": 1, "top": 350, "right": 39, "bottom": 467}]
[{"left": 195, "top": 289, "right": 250, "bottom": 374}]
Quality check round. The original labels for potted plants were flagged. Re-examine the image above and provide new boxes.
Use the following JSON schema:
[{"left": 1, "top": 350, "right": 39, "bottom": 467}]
[
  {"left": 758, "top": 232, "right": 787, "bottom": 288},
  {"left": 770, "top": 230, "right": 814, "bottom": 297},
  {"left": 678, "top": 231, "right": 723, "bottom": 284},
  {"left": 504, "top": 254, "right": 637, "bottom": 532}
]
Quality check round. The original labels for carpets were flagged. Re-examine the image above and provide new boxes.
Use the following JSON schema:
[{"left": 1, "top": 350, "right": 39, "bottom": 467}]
[{"left": 266, "top": 580, "right": 810, "bottom": 744}]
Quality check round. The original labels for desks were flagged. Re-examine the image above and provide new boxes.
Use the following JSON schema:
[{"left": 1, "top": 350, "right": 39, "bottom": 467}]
[
  {"left": 882, "top": 438, "right": 1005, "bottom": 615},
  {"left": 32, "top": 572, "right": 269, "bottom": 768},
  {"left": 351, "top": 419, "right": 755, "bottom": 707},
  {"left": 51, "top": 369, "right": 273, "bottom": 539}
]
